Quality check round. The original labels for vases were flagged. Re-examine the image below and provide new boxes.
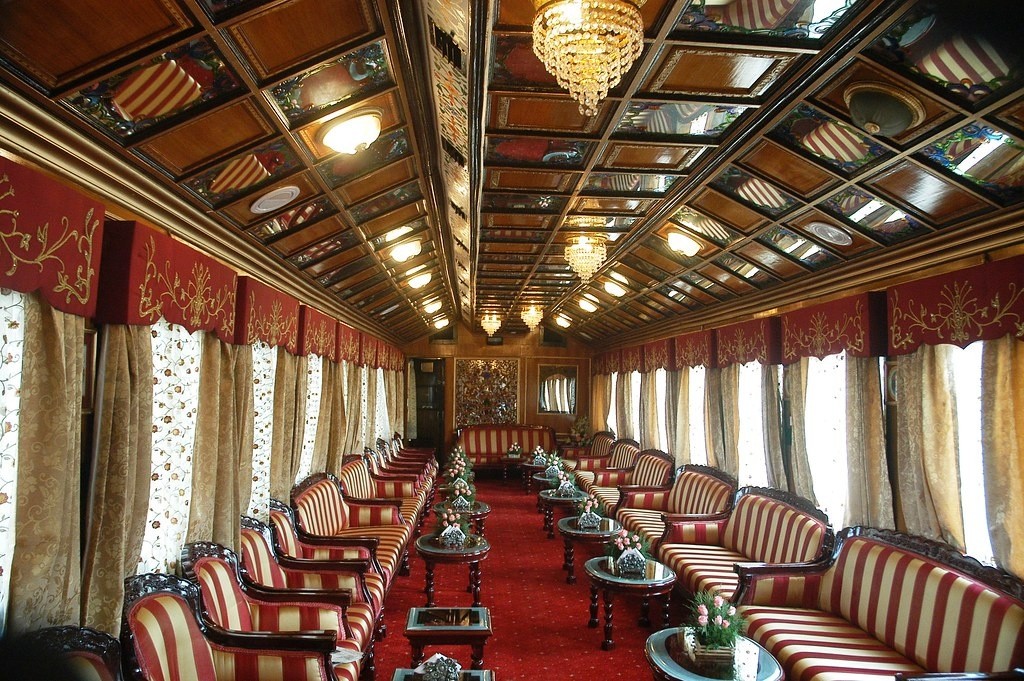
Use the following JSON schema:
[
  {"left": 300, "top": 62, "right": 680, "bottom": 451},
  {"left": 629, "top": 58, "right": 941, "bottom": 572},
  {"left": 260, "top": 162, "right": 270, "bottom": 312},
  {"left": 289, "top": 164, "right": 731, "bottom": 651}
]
[
  {"left": 580, "top": 516, "right": 600, "bottom": 531},
  {"left": 452, "top": 497, "right": 469, "bottom": 510},
  {"left": 560, "top": 487, "right": 574, "bottom": 497},
  {"left": 509, "top": 454, "right": 520, "bottom": 459},
  {"left": 618, "top": 556, "right": 646, "bottom": 577},
  {"left": 452, "top": 478, "right": 467, "bottom": 486},
  {"left": 441, "top": 528, "right": 465, "bottom": 547},
  {"left": 690, "top": 636, "right": 736, "bottom": 658},
  {"left": 546, "top": 468, "right": 558, "bottom": 477},
  {"left": 533, "top": 459, "right": 545, "bottom": 466}
]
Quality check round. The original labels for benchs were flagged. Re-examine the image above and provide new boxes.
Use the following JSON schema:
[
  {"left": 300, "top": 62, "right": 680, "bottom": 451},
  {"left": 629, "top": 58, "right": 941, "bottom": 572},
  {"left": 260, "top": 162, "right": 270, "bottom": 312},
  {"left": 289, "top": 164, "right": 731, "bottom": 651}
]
[
  {"left": 290, "top": 472, "right": 410, "bottom": 638},
  {"left": 573, "top": 439, "right": 640, "bottom": 492},
  {"left": 589, "top": 448, "right": 676, "bottom": 518},
  {"left": 376, "top": 431, "right": 438, "bottom": 513},
  {"left": 614, "top": 464, "right": 738, "bottom": 559},
  {"left": 656, "top": 486, "right": 835, "bottom": 613},
  {"left": 341, "top": 454, "right": 421, "bottom": 575},
  {"left": 454, "top": 423, "right": 558, "bottom": 473},
  {"left": 734, "top": 525, "right": 1024, "bottom": 681},
  {"left": 561, "top": 432, "right": 616, "bottom": 473},
  {"left": 363, "top": 446, "right": 430, "bottom": 535}
]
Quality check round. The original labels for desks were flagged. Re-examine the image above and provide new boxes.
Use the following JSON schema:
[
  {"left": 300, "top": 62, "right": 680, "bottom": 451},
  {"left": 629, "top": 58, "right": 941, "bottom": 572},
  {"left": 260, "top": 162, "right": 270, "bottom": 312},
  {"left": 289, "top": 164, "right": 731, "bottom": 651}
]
[
  {"left": 439, "top": 462, "right": 476, "bottom": 502},
  {"left": 583, "top": 556, "right": 678, "bottom": 652},
  {"left": 391, "top": 668, "right": 495, "bottom": 681},
  {"left": 499, "top": 456, "right": 526, "bottom": 484},
  {"left": 522, "top": 460, "right": 546, "bottom": 495},
  {"left": 432, "top": 502, "right": 491, "bottom": 537},
  {"left": 403, "top": 605, "right": 493, "bottom": 670},
  {"left": 539, "top": 489, "right": 589, "bottom": 539},
  {"left": 532, "top": 472, "right": 564, "bottom": 513},
  {"left": 557, "top": 516, "right": 623, "bottom": 584},
  {"left": 414, "top": 534, "right": 491, "bottom": 607},
  {"left": 644, "top": 627, "right": 785, "bottom": 681}
]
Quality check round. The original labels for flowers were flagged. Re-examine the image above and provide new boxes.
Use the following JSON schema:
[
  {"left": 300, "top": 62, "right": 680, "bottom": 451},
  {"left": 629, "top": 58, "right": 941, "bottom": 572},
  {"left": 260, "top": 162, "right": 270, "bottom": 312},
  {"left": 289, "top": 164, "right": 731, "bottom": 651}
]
[
  {"left": 448, "top": 446, "right": 470, "bottom": 462},
  {"left": 604, "top": 529, "right": 652, "bottom": 561},
  {"left": 577, "top": 493, "right": 605, "bottom": 517},
  {"left": 451, "top": 483, "right": 475, "bottom": 502},
  {"left": 682, "top": 589, "right": 748, "bottom": 652},
  {"left": 532, "top": 445, "right": 547, "bottom": 461},
  {"left": 548, "top": 471, "right": 579, "bottom": 496},
  {"left": 545, "top": 449, "right": 565, "bottom": 472},
  {"left": 448, "top": 468, "right": 468, "bottom": 482},
  {"left": 436, "top": 507, "right": 472, "bottom": 533},
  {"left": 506, "top": 442, "right": 522, "bottom": 455},
  {"left": 449, "top": 455, "right": 471, "bottom": 471}
]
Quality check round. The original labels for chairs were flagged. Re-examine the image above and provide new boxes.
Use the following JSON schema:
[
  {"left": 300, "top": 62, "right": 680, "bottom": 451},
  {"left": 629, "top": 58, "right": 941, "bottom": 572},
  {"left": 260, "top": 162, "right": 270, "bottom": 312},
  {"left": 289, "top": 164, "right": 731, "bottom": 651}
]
[
  {"left": 0, "top": 626, "right": 125, "bottom": 681},
  {"left": 240, "top": 515, "right": 376, "bottom": 681},
  {"left": 181, "top": 542, "right": 366, "bottom": 681},
  {"left": 120, "top": 573, "right": 336, "bottom": 681},
  {"left": 267, "top": 500, "right": 383, "bottom": 662}
]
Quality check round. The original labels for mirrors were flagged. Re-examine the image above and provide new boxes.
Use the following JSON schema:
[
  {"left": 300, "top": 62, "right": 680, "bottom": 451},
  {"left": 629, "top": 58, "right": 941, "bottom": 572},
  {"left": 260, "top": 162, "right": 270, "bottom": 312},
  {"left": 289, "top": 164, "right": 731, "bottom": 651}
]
[
  {"left": 262, "top": 39, "right": 398, "bottom": 130},
  {"left": 763, "top": 103, "right": 899, "bottom": 180},
  {"left": 707, "top": 161, "right": 805, "bottom": 223},
  {"left": 316, "top": 205, "right": 747, "bottom": 321},
  {"left": 283, "top": 230, "right": 363, "bottom": 271},
  {"left": 480, "top": 192, "right": 571, "bottom": 214},
  {"left": 177, "top": 136, "right": 307, "bottom": 210},
  {"left": 755, "top": 227, "right": 842, "bottom": 274},
  {"left": 346, "top": 181, "right": 422, "bottom": 225},
  {"left": 612, "top": 98, "right": 751, "bottom": 144},
  {"left": 247, "top": 195, "right": 340, "bottom": 246},
  {"left": 577, "top": 172, "right": 687, "bottom": 202},
  {"left": 665, "top": 0, "right": 873, "bottom": 50},
  {"left": 861, "top": 0, "right": 1024, "bottom": 115},
  {"left": 814, "top": 185, "right": 934, "bottom": 249},
  {"left": 314, "top": 129, "right": 412, "bottom": 192},
  {"left": 483, "top": 136, "right": 598, "bottom": 171},
  {"left": 709, "top": 252, "right": 782, "bottom": 294},
  {"left": 59, "top": 33, "right": 250, "bottom": 148},
  {"left": 912, "top": 121, "right": 1024, "bottom": 208}
]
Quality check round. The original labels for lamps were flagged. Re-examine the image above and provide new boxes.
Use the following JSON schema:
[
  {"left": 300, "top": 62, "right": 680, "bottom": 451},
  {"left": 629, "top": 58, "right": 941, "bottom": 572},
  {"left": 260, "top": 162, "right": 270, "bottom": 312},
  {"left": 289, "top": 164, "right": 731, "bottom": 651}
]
[
  {"left": 563, "top": 233, "right": 609, "bottom": 285},
  {"left": 843, "top": 81, "right": 926, "bottom": 137},
  {"left": 665, "top": 227, "right": 707, "bottom": 257},
  {"left": 405, "top": 269, "right": 432, "bottom": 289},
  {"left": 577, "top": 298, "right": 600, "bottom": 313},
  {"left": 556, "top": 316, "right": 572, "bottom": 328},
  {"left": 604, "top": 280, "right": 627, "bottom": 298},
  {"left": 481, "top": 315, "right": 501, "bottom": 337},
  {"left": 433, "top": 318, "right": 449, "bottom": 329},
  {"left": 315, "top": 105, "right": 386, "bottom": 155},
  {"left": 531, "top": 0, "right": 648, "bottom": 118},
  {"left": 387, "top": 235, "right": 422, "bottom": 263},
  {"left": 424, "top": 301, "right": 442, "bottom": 313}
]
